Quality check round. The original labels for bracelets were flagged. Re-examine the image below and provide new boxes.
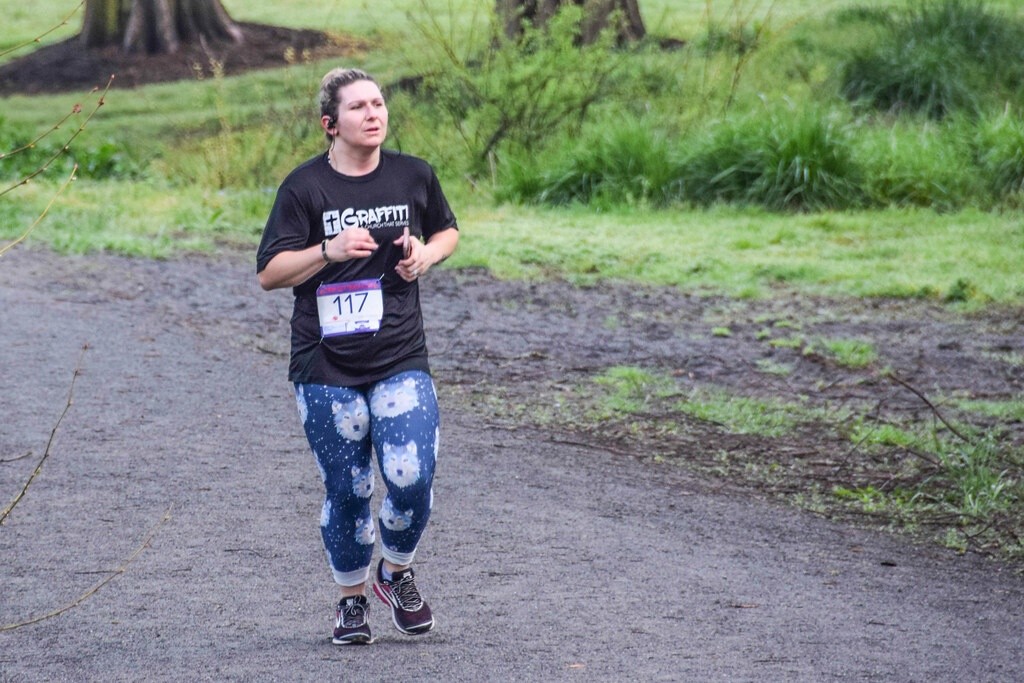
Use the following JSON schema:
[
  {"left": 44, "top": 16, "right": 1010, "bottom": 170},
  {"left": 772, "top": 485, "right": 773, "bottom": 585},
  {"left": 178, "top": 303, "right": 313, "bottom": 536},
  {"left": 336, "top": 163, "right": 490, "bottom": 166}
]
[{"left": 321, "top": 239, "right": 332, "bottom": 264}]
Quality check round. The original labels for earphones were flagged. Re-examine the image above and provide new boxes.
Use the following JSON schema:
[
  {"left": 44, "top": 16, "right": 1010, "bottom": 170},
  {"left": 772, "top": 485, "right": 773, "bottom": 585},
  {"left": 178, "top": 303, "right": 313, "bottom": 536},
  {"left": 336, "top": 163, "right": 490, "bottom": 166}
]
[{"left": 326, "top": 115, "right": 337, "bottom": 129}]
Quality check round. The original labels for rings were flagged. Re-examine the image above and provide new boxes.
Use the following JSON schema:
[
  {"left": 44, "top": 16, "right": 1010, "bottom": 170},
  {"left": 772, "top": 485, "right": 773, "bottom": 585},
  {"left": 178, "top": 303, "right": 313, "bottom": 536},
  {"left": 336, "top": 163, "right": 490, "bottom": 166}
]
[{"left": 412, "top": 269, "right": 418, "bottom": 276}]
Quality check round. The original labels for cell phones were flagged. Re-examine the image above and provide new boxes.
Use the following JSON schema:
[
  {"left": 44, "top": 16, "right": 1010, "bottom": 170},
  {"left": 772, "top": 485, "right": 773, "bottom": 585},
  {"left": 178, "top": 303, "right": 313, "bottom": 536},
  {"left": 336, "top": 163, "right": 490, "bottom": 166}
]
[{"left": 403, "top": 226, "right": 411, "bottom": 260}]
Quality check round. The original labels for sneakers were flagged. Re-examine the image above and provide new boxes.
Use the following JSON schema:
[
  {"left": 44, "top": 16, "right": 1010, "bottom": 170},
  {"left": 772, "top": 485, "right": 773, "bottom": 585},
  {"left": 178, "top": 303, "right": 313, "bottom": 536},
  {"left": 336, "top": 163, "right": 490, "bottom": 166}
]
[
  {"left": 373, "top": 556, "right": 434, "bottom": 635},
  {"left": 332, "top": 596, "right": 373, "bottom": 646}
]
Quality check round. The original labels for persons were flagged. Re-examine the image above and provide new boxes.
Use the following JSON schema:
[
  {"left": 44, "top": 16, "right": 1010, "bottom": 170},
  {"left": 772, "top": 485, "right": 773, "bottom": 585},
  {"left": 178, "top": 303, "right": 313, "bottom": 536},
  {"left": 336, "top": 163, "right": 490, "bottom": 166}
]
[{"left": 255, "top": 66, "right": 461, "bottom": 647}]
